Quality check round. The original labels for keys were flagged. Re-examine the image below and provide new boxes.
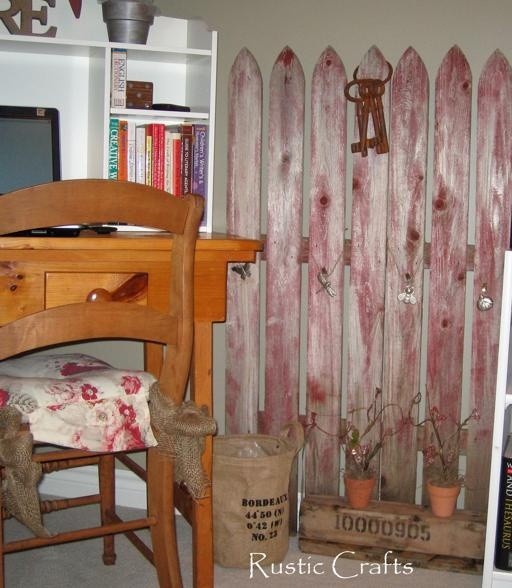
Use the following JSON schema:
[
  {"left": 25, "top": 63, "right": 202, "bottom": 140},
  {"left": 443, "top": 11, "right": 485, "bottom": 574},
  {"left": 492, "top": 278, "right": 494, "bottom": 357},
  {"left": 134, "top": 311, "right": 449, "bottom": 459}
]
[{"left": 344, "top": 77, "right": 389, "bottom": 158}]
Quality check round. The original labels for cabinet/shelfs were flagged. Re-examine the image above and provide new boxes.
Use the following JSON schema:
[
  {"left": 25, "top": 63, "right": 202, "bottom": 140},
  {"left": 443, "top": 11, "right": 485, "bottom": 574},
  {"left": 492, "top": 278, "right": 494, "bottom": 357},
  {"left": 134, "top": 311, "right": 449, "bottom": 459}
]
[
  {"left": 483, "top": 249, "right": 512, "bottom": 555},
  {"left": 0, "top": 1, "right": 219, "bottom": 233}
]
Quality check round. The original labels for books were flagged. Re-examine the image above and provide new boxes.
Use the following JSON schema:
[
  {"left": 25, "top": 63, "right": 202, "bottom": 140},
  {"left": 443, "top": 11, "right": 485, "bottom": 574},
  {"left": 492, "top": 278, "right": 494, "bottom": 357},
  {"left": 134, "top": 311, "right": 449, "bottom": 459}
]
[
  {"left": 110, "top": 48, "right": 127, "bottom": 109},
  {"left": 107, "top": 118, "right": 213, "bottom": 227}
]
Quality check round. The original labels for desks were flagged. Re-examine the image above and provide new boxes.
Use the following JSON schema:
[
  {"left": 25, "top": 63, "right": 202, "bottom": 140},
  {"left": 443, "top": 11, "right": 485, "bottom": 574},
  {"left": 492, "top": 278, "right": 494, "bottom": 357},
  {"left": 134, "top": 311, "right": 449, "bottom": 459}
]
[{"left": 0, "top": 230, "right": 262, "bottom": 588}]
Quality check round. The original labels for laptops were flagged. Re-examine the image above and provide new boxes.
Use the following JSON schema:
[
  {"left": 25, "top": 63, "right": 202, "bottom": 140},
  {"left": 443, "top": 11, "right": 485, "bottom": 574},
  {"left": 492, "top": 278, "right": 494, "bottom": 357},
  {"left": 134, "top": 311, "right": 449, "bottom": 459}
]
[{"left": 0, "top": 106, "right": 119, "bottom": 235}]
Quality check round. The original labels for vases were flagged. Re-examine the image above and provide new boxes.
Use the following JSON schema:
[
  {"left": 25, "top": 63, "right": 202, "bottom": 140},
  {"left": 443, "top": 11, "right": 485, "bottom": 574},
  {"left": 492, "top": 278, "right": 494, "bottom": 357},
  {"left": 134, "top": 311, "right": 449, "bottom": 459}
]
[
  {"left": 425, "top": 477, "right": 462, "bottom": 517},
  {"left": 344, "top": 471, "right": 375, "bottom": 510}
]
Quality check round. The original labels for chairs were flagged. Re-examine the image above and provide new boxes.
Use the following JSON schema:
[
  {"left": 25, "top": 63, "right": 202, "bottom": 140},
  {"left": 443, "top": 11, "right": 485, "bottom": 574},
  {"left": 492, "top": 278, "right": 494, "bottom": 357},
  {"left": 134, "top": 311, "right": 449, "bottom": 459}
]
[{"left": 0, "top": 181, "right": 208, "bottom": 588}]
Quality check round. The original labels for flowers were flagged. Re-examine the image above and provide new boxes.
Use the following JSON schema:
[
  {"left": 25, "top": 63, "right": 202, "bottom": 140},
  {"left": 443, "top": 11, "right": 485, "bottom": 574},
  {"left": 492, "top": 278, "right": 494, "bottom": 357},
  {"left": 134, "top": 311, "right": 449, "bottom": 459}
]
[
  {"left": 309, "top": 387, "right": 409, "bottom": 477},
  {"left": 402, "top": 392, "right": 484, "bottom": 484}
]
